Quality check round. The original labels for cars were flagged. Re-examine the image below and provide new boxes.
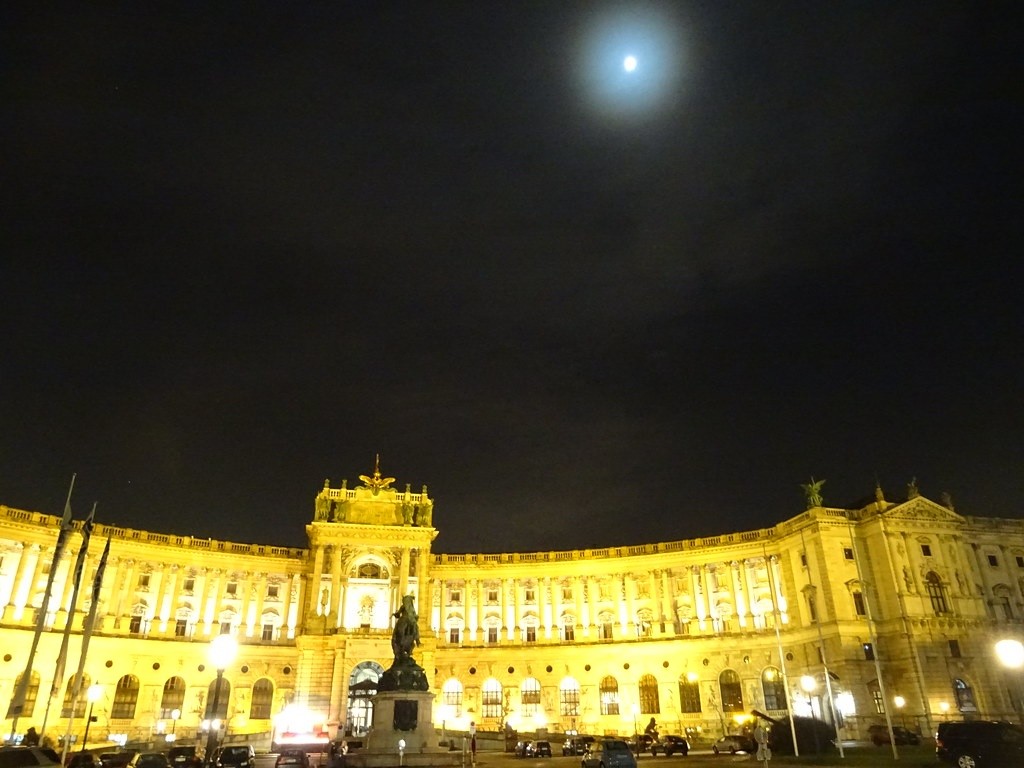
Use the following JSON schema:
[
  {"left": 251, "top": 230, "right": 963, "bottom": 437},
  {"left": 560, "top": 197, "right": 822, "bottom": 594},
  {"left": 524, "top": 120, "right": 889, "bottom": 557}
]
[
  {"left": 626, "top": 734, "right": 657, "bottom": 758},
  {"left": 935, "top": 719, "right": 1023, "bottom": 768},
  {"left": 711, "top": 733, "right": 756, "bottom": 754},
  {"left": 274, "top": 749, "right": 311, "bottom": 768},
  {"left": 168, "top": 747, "right": 207, "bottom": 768},
  {"left": 562, "top": 738, "right": 588, "bottom": 756},
  {"left": 526, "top": 741, "right": 553, "bottom": 759},
  {"left": 210, "top": 746, "right": 225, "bottom": 768},
  {"left": 216, "top": 746, "right": 256, "bottom": 768},
  {"left": 579, "top": 741, "right": 638, "bottom": 768},
  {"left": 868, "top": 723, "right": 920, "bottom": 747},
  {"left": 514, "top": 740, "right": 534, "bottom": 758},
  {"left": 650, "top": 735, "right": 689, "bottom": 758}
]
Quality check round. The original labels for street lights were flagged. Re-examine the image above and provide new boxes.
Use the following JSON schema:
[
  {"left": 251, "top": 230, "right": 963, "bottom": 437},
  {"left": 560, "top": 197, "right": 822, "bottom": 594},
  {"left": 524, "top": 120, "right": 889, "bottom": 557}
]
[{"left": 81, "top": 633, "right": 240, "bottom": 768}]
